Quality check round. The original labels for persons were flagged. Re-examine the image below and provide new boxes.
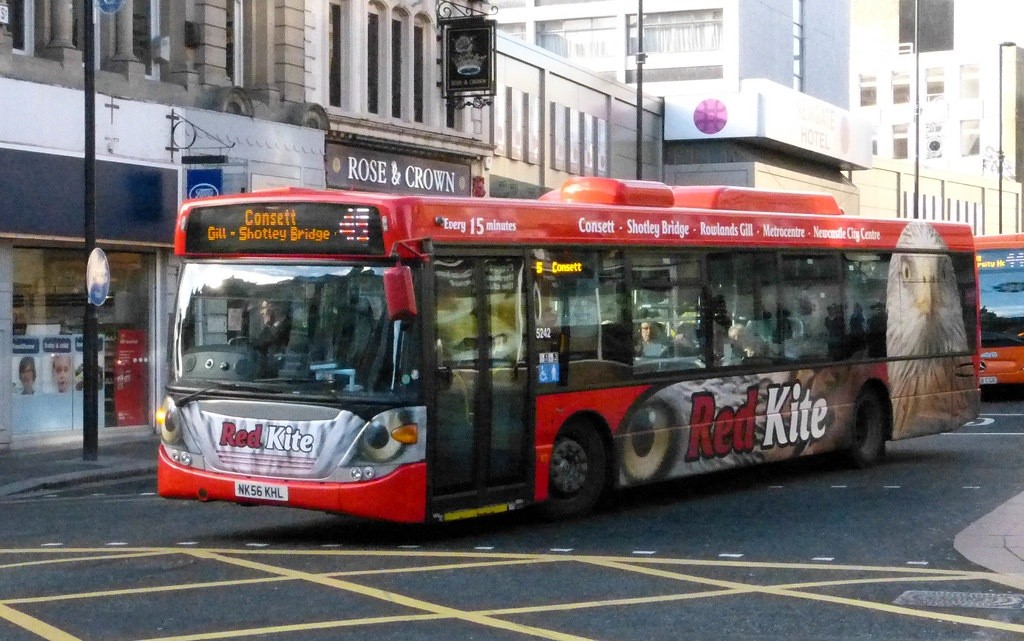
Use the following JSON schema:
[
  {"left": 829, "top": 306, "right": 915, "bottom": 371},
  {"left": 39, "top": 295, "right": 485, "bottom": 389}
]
[
  {"left": 631, "top": 309, "right": 766, "bottom": 358},
  {"left": 251, "top": 296, "right": 286, "bottom": 376},
  {"left": 44, "top": 355, "right": 72, "bottom": 394},
  {"left": 17, "top": 356, "right": 38, "bottom": 395}
]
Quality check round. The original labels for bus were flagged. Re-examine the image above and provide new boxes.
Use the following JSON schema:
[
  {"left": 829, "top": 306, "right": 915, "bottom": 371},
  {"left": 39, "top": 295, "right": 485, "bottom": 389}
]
[
  {"left": 156, "top": 177, "right": 982, "bottom": 523},
  {"left": 974, "top": 233, "right": 1024, "bottom": 384}
]
